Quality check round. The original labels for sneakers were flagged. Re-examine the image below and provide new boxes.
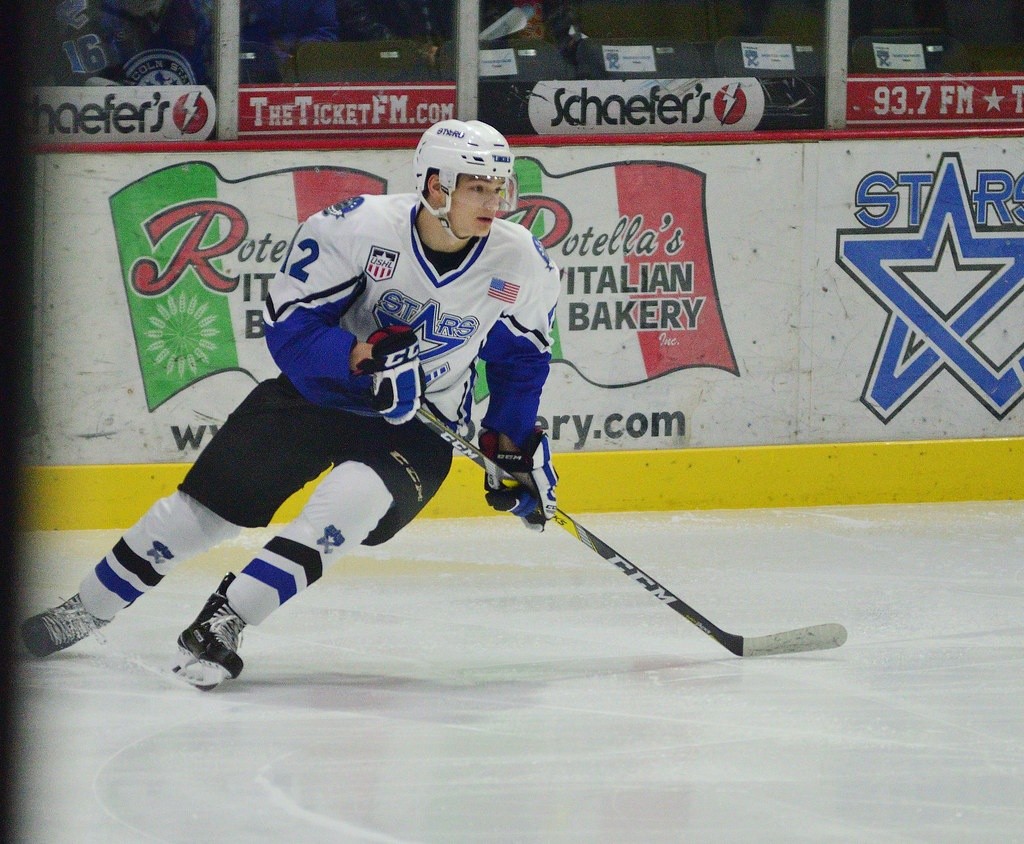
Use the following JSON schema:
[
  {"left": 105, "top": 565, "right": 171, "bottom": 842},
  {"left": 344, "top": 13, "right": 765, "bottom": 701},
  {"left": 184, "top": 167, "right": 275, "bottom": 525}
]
[
  {"left": 21, "top": 595, "right": 116, "bottom": 659},
  {"left": 175, "top": 572, "right": 243, "bottom": 693}
]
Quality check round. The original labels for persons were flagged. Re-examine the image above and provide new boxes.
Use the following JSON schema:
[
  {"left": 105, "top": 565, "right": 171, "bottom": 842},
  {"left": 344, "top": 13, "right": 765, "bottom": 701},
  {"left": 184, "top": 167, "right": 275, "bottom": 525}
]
[
  {"left": 14, "top": 118, "right": 559, "bottom": 689},
  {"left": 55, "top": 0, "right": 603, "bottom": 135}
]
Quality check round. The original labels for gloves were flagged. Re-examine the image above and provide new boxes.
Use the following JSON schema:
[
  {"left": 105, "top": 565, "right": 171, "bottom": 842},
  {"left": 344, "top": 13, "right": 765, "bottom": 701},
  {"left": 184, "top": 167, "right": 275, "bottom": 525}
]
[
  {"left": 354, "top": 327, "right": 424, "bottom": 426},
  {"left": 477, "top": 431, "right": 563, "bottom": 533}
]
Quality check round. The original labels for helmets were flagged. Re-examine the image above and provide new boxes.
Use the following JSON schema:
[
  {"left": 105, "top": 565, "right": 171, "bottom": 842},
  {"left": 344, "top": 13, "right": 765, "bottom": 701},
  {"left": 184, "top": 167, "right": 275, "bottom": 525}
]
[{"left": 414, "top": 120, "right": 514, "bottom": 200}]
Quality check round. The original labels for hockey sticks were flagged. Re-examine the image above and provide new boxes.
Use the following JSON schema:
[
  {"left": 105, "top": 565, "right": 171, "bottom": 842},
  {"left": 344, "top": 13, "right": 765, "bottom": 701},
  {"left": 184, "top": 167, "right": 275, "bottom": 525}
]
[{"left": 411, "top": 407, "right": 850, "bottom": 662}]
